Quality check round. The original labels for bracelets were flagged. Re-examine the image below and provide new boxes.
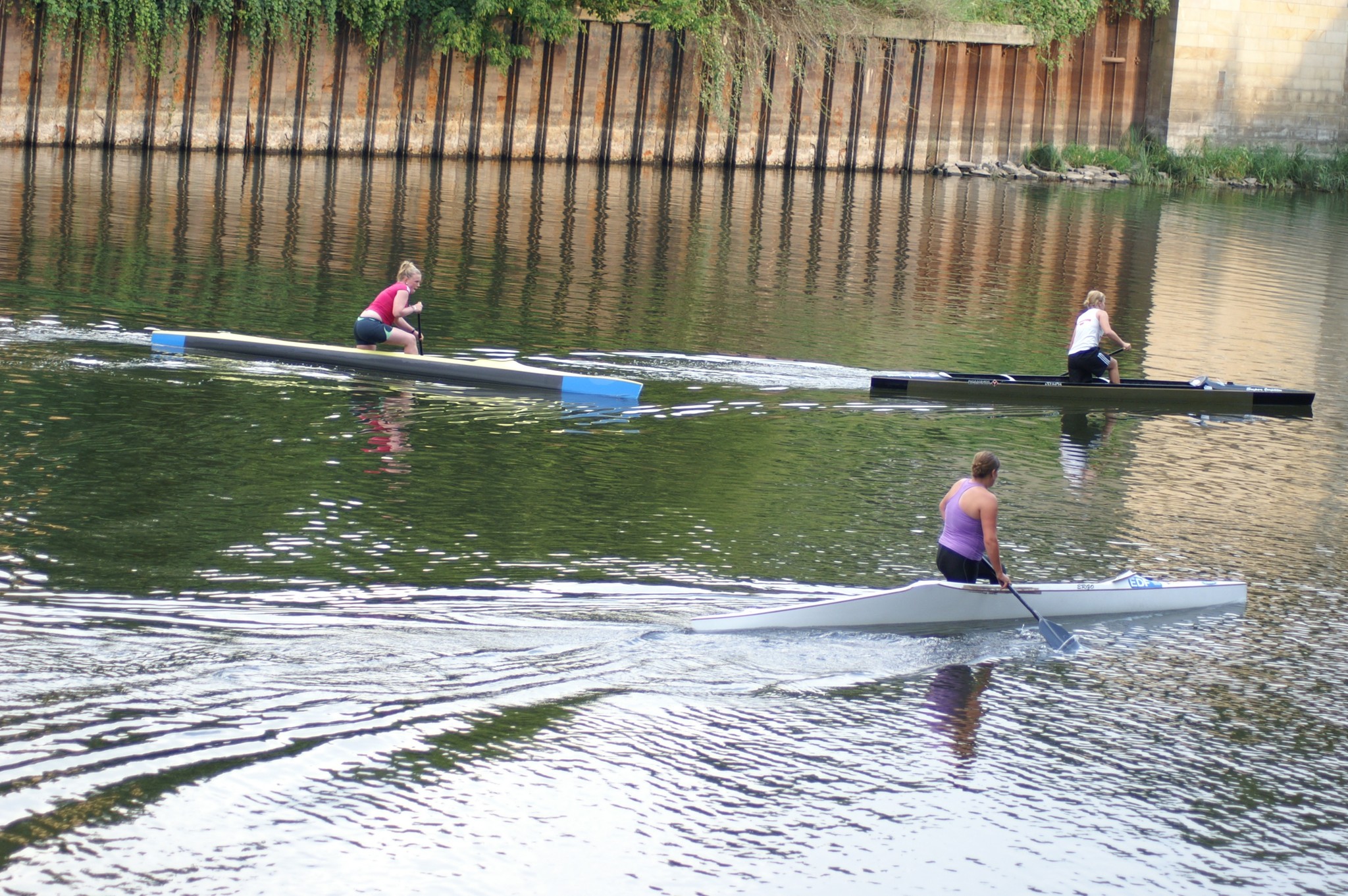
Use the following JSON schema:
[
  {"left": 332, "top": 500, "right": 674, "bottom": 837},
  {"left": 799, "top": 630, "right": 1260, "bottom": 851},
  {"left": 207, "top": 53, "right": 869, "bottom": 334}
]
[{"left": 410, "top": 327, "right": 416, "bottom": 334}]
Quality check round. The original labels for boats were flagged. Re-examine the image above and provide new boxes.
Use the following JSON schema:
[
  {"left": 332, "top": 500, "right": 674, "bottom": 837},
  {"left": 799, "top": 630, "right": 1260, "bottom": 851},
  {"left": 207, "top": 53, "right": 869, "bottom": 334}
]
[
  {"left": 152, "top": 326, "right": 644, "bottom": 399},
  {"left": 869, "top": 372, "right": 1317, "bottom": 414},
  {"left": 681, "top": 578, "right": 1250, "bottom": 633}
]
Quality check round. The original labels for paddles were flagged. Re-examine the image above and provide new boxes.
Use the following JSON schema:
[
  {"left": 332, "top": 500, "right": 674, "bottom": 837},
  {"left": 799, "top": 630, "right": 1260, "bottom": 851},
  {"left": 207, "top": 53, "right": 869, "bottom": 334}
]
[
  {"left": 1060, "top": 347, "right": 1126, "bottom": 376},
  {"left": 981, "top": 554, "right": 1080, "bottom": 652},
  {"left": 417, "top": 301, "right": 423, "bottom": 355}
]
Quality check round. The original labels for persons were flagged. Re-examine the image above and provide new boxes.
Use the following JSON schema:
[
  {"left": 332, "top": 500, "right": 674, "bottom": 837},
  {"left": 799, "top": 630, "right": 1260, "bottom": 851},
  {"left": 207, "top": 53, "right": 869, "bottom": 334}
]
[
  {"left": 353, "top": 260, "right": 424, "bottom": 356},
  {"left": 935, "top": 451, "right": 1014, "bottom": 589},
  {"left": 1068, "top": 290, "right": 1131, "bottom": 385}
]
